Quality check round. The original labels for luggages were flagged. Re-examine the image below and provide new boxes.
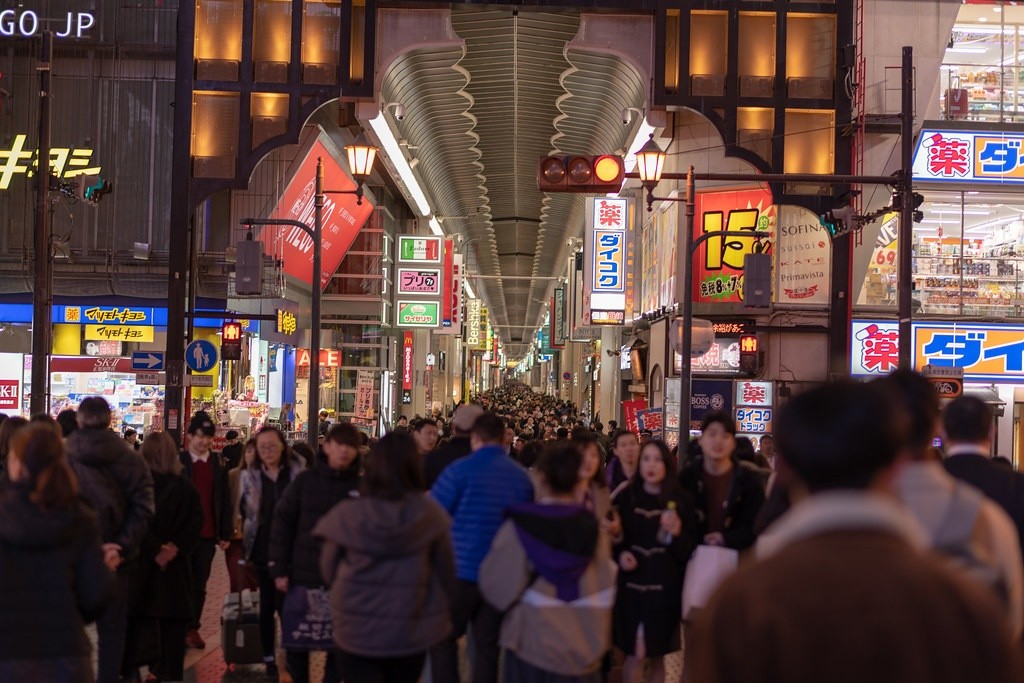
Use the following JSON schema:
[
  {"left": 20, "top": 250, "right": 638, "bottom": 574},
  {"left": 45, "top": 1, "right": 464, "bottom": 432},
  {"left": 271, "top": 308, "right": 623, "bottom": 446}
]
[{"left": 218, "top": 560, "right": 264, "bottom": 671}]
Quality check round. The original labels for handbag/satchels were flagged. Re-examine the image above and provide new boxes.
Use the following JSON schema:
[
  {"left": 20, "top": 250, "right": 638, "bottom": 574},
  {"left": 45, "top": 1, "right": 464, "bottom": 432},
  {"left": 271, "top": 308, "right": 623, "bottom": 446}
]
[
  {"left": 279, "top": 585, "right": 338, "bottom": 651},
  {"left": 124, "top": 545, "right": 140, "bottom": 562},
  {"left": 680, "top": 542, "right": 741, "bottom": 621}
]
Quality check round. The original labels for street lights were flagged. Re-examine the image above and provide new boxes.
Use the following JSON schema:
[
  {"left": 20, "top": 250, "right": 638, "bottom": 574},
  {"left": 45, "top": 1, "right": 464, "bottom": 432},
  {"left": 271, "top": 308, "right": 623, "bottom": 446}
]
[
  {"left": 308, "top": 142, "right": 381, "bottom": 454},
  {"left": 634, "top": 134, "right": 694, "bottom": 472}
]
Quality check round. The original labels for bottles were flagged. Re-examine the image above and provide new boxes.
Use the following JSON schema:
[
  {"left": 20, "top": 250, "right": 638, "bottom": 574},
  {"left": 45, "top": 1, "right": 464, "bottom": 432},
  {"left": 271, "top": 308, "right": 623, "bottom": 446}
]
[
  {"left": 658, "top": 500, "right": 677, "bottom": 543},
  {"left": 927, "top": 277, "right": 978, "bottom": 288}
]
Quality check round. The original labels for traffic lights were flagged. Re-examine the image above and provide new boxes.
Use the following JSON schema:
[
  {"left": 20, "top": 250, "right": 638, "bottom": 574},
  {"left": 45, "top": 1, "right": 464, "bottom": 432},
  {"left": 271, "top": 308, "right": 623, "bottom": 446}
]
[
  {"left": 220, "top": 322, "right": 242, "bottom": 360},
  {"left": 738, "top": 334, "right": 759, "bottom": 372},
  {"left": 819, "top": 204, "right": 856, "bottom": 239},
  {"left": 537, "top": 155, "right": 625, "bottom": 192},
  {"left": 79, "top": 172, "right": 112, "bottom": 208}
]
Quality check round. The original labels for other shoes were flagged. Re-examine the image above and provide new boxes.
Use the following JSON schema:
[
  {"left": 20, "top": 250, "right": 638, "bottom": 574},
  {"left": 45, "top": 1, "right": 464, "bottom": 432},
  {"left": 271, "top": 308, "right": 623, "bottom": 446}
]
[
  {"left": 186, "top": 629, "right": 207, "bottom": 649},
  {"left": 263, "top": 661, "right": 279, "bottom": 680},
  {"left": 145, "top": 672, "right": 159, "bottom": 683}
]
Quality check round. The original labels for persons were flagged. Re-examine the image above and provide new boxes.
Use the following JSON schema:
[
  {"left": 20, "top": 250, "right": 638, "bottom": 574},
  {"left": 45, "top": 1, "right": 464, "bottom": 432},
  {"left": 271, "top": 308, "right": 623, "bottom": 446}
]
[
  {"left": 411, "top": 380, "right": 619, "bottom": 459},
  {"left": 397, "top": 415, "right": 407, "bottom": 429},
  {"left": 318, "top": 410, "right": 328, "bottom": 435},
  {"left": 475, "top": 410, "right": 777, "bottom": 683},
  {"left": 683, "top": 367, "right": 1024, "bottom": 683},
  {"left": 0, "top": 397, "right": 232, "bottom": 683},
  {"left": 218, "top": 421, "right": 536, "bottom": 683}
]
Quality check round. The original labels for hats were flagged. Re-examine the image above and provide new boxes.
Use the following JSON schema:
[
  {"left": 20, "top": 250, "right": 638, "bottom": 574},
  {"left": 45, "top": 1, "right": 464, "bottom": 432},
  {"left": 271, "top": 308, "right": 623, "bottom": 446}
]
[
  {"left": 188, "top": 410, "right": 215, "bottom": 437},
  {"left": 454, "top": 408, "right": 482, "bottom": 430}
]
